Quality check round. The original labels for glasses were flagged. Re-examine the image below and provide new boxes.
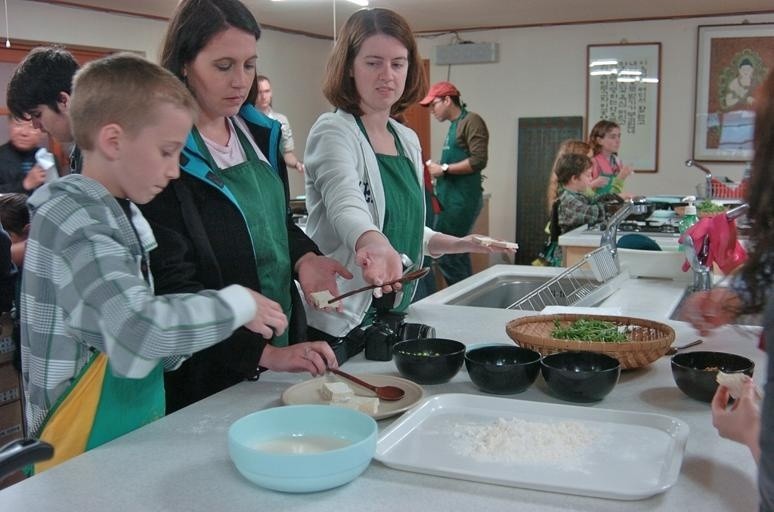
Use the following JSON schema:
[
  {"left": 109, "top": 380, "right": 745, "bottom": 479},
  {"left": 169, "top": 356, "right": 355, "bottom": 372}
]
[{"left": 10, "top": 120, "right": 41, "bottom": 136}]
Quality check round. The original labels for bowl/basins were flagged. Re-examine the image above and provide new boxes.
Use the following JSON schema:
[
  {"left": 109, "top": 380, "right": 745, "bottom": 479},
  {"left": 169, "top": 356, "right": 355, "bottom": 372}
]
[
  {"left": 539, "top": 349, "right": 620, "bottom": 402},
  {"left": 225, "top": 403, "right": 378, "bottom": 494},
  {"left": 671, "top": 351, "right": 756, "bottom": 404},
  {"left": 394, "top": 337, "right": 467, "bottom": 384},
  {"left": 464, "top": 345, "right": 542, "bottom": 396}
]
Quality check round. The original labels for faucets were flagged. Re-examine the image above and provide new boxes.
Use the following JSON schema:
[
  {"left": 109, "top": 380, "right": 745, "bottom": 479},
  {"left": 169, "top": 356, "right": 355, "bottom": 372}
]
[
  {"left": 684, "top": 159, "right": 713, "bottom": 201},
  {"left": 599, "top": 196, "right": 648, "bottom": 275},
  {"left": 692, "top": 203, "right": 753, "bottom": 294}
]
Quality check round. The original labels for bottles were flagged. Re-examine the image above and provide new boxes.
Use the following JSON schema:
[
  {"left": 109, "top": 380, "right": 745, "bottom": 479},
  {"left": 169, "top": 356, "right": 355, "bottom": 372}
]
[{"left": 363, "top": 318, "right": 393, "bottom": 361}]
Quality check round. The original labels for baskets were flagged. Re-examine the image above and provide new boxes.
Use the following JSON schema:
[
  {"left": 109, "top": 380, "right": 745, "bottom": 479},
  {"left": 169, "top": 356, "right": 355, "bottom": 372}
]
[
  {"left": 507, "top": 243, "right": 619, "bottom": 310},
  {"left": 711, "top": 177, "right": 749, "bottom": 199},
  {"left": 505, "top": 310, "right": 677, "bottom": 373}
]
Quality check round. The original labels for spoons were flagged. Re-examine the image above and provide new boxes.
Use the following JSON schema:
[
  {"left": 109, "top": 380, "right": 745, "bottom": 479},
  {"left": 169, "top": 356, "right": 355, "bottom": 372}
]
[
  {"left": 328, "top": 364, "right": 404, "bottom": 404},
  {"left": 666, "top": 337, "right": 705, "bottom": 356},
  {"left": 327, "top": 263, "right": 429, "bottom": 306}
]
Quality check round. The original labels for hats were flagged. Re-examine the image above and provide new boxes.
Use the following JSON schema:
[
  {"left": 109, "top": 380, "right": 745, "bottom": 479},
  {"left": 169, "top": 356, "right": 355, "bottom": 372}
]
[{"left": 419, "top": 81, "right": 460, "bottom": 107}]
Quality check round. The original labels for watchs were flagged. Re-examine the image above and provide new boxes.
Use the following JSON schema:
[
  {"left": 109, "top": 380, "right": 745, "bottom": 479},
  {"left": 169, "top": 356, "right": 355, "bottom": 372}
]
[{"left": 441, "top": 162, "right": 450, "bottom": 176}]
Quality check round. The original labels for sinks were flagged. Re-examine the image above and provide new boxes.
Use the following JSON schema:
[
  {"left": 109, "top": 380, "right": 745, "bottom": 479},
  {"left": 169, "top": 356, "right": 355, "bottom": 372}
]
[
  {"left": 442, "top": 272, "right": 621, "bottom": 312},
  {"left": 668, "top": 286, "right": 765, "bottom": 328}
]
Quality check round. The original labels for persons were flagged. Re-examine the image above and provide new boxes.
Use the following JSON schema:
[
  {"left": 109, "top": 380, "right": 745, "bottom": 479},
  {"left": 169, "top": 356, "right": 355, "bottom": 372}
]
[
  {"left": 709, "top": 287, "right": 774, "bottom": 511},
  {"left": 421, "top": 82, "right": 489, "bottom": 284},
  {"left": 296, "top": 6, "right": 518, "bottom": 369},
  {"left": 537, "top": 121, "right": 634, "bottom": 267},
  {"left": 679, "top": 66, "right": 772, "bottom": 340},
  {"left": 723, "top": 60, "right": 759, "bottom": 110}
]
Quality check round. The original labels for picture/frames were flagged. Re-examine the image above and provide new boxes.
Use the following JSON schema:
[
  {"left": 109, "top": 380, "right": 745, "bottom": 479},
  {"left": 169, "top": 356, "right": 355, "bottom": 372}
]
[
  {"left": 586, "top": 42, "right": 662, "bottom": 174},
  {"left": 694, "top": 24, "right": 774, "bottom": 163}
]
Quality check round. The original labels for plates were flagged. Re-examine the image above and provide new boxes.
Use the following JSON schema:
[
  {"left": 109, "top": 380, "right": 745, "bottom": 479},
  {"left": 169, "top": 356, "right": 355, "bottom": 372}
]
[
  {"left": 282, "top": 374, "right": 425, "bottom": 419},
  {"left": 617, "top": 234, "right": 663, "bottom": 251}
]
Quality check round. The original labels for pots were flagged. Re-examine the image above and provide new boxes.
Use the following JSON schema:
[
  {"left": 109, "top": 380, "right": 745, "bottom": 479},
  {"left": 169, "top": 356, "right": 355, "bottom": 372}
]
[{"left": 605, "top": 201, "right": 656, "bottom": 220}]
